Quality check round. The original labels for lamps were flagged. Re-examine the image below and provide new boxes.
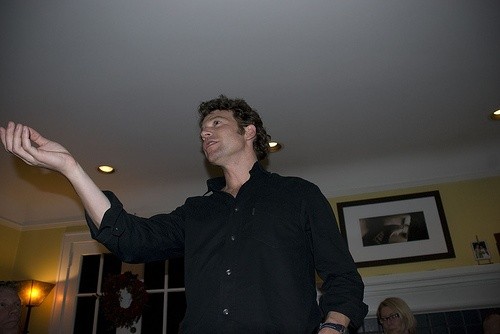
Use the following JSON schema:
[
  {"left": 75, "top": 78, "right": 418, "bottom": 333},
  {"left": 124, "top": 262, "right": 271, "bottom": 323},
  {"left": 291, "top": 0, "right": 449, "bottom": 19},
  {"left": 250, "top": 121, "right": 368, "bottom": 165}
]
[{"left": 17, "top": 280, "right": 56, "bottom": 334}]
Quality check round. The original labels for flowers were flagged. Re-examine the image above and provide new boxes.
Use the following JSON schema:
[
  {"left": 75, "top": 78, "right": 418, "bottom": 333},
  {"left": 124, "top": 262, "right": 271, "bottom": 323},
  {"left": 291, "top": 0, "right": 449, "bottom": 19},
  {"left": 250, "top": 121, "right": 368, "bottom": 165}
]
[{"left": 92, "top": 271, "right": 149, "bottom": 330}]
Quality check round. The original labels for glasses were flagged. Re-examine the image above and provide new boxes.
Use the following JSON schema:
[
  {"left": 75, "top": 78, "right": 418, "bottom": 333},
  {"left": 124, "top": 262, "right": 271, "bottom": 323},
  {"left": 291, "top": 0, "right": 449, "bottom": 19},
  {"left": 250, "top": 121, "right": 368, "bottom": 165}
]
[{"left": 379, "top": 313, "right": 400, "bottom": 324}]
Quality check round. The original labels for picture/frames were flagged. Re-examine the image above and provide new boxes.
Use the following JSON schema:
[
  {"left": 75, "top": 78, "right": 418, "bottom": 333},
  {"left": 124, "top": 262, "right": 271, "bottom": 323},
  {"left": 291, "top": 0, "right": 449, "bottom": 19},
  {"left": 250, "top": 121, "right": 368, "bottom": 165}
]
[
  {"left": 472, "top": 240, "right": 491, "bottom": 260},
  {"left": 337, "top": 190, "right": 456, "bottom": 268}
]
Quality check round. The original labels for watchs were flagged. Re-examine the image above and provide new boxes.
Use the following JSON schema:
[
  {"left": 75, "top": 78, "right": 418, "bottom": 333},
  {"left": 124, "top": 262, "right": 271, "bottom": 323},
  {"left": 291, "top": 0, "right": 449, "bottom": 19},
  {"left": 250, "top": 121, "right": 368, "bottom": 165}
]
[{"left": 319, "top": 322, "right": 346, "bottom": 334}]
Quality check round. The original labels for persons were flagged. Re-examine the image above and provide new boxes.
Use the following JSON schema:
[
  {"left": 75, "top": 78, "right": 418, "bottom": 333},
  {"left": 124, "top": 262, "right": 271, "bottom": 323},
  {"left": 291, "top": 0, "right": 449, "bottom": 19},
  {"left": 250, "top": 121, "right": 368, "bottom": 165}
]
[
  {"left": 375, "top": 297, "right": 417, "bottom": 334},
  {"left": 475, "top": 245, "right": 488, "bottom": 258},
  {"left": 0, "top": 95, "right": 369, "bottom": 334},
  {"left": 483, "top": 314, "right": 500, "bottom": 334}
]
